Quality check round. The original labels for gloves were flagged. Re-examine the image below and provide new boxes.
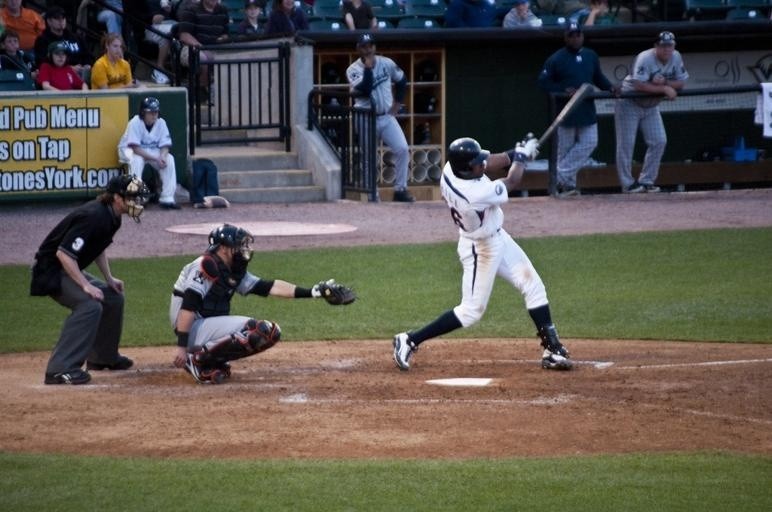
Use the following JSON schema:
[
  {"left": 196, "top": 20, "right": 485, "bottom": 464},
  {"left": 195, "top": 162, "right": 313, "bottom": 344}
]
[{"left": 514, "top": 132, "right": 541, "bottom": 161}]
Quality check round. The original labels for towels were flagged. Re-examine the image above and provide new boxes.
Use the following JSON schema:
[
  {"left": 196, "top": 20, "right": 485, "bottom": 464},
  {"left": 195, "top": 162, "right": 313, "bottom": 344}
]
[{"left": 752, "top": 82, "right": 771, "bottom": 139}]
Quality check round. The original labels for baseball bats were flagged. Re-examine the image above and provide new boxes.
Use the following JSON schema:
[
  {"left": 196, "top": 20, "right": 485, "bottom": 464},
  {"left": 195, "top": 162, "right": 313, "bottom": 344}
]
[{"left": 536, "top": 82, "right": 593, "bottom": 150}]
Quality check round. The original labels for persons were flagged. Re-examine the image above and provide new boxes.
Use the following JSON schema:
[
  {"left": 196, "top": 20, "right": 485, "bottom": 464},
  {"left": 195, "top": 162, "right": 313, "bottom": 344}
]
[
  {"left": 615, "top": 31, "right": 690, "bottom": 193},
  {"left": 392, "top": 132, "right": 574, "bottom": 373},
  {"left": 184, "top": 160, "right": 230, "bottom": 210},
  {"left": 1, "top": 0, "right": 177, "bottom": 91},
  {"left": 342, "top": 0, "right": 377, "bottom": 30},
  {"left": 117, "top": 96, "right": 181, "bottom": 211},
  {"left": 168, "top": 223, "right": 354, "bottom": 384},
  {"left": 538, "top": 22, "right": 620, "bottom": 198},
  {"left": 29, "top": 174, "right": 153, "bottom": 386},
  {"left": 446, "top": 0, "right": 624, "bottom": 29},
  {"left": 345, "top": 33, "right": 416, "bottom": 203},
  {"left": 179, "top": 0, "right": 313, "bottom": 68}
]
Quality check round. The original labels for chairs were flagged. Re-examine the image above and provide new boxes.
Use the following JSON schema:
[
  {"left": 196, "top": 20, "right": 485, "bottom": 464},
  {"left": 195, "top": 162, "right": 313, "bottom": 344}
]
[{"left": 1, "top": 1, "right": 772, "bottom": 103}]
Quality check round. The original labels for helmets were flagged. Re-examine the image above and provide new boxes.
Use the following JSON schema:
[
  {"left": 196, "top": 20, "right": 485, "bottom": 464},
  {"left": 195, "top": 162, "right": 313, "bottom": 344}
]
[
  {"left": 138, "top": 96, "right": 161, "bottom": 119},
  {"left": 106, "top": 174, "right": 151, "bottom": 218},
  {"left": 206, "top": 223, "right": 249, "bottom": 254},
  {"left": 447, "top": 136, "right": 490, "bottom": 179}
]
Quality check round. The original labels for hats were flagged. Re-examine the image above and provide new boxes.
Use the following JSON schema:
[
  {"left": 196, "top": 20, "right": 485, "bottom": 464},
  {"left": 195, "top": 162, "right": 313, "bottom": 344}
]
[
  {"left": 515, "top": 0, "right": 528, "bottom": 6},
  {"left": 48, "top": 42, "right": 70, "bottom": 57},
  {"left": 656, "top": 30, "right": 675, "bottom": 46},
  {"left": 46, "top": 6, "right": 66, "bottom": 17},
  {"left": 359, "top": 32, "right": 375, "bottom": 47},
  {"left": 245, "top": 0, "right": 261, "bottom": 6},
  {"left": 564, "top": 22, "right": 582, "bottom": 38}
]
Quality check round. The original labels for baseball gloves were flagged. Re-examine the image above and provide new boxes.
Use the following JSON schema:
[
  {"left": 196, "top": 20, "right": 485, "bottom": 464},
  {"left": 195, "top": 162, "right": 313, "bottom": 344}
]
[{"left": 311, "top": 279, "right": 355, "bottom": 304}]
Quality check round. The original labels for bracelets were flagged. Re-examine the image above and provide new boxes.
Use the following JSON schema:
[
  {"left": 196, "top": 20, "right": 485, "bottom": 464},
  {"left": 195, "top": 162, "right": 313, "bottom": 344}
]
[
  {"left": 512, "top": 152, "right": 530, "bottom": 167},
  {"left": 295, "top": 286, "right": 312, "bottom": 299},
  {"left": 504, "top": 148, "right": 516, "bottom": 164},
  {"left": 176, "top": 331, "right": 188, "bottom": 348},
  {"left": 664, "top": 78, "right": 668, "bottom": 85}
]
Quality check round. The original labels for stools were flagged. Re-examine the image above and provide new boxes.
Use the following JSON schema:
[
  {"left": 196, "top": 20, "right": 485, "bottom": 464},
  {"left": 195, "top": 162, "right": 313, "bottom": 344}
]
[{"left": 118, "top": 158, "right": 161, "bottom": 208}]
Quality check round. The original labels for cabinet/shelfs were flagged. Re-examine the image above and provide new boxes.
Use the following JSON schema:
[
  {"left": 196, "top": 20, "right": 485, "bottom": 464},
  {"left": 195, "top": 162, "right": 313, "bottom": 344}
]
[{"left": 309, "top": 45, "right": 451, "bottom": 204}]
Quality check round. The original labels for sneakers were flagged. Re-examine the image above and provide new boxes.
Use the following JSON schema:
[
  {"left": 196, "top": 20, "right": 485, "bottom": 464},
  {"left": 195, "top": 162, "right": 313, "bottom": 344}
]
[
  {"left": 85, "top": 353, "right": 134, "bottom": 371},
  {"left": 393, "top": 188, "right": 417, "bottom": 202},
  {"left": 159, "top": 199, "right": 181, "bottom": 211},
  {"left": 44, "top": 368, "right": 92, "bottom": 385},
  {"left": 540, "top": 344, "right": 579, "bottom": 370},
  {"left": 623, "top": 180, "right": 661, "bottom": 194},
  {"left": 555, "top": 183, "right": 579, "bottom": 197},
  {"left": 184, "top": 350, "right": 232, "bottom": 385},
  {"left": 391, "top": 330, "right": 416, "bottom": 370}
]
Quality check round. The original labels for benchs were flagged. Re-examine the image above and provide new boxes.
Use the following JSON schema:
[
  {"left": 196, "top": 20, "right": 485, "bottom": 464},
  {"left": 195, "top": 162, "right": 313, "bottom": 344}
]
[{"left": 476, "top": 153, "right": 771, "bottom": 200}]
